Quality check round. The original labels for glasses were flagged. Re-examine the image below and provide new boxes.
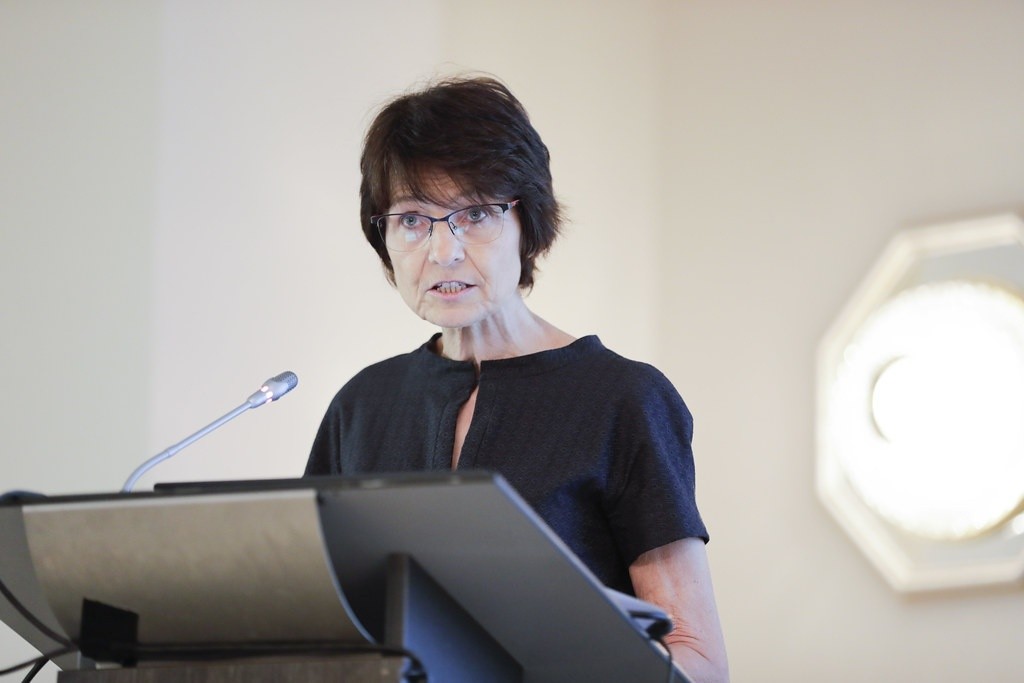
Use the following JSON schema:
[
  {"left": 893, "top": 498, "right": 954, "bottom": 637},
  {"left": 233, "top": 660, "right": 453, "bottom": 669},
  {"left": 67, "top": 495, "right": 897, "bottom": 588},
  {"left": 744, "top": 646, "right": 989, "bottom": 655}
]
[{"left": 371, "top": 199, "right": 520, "bottom": 252}]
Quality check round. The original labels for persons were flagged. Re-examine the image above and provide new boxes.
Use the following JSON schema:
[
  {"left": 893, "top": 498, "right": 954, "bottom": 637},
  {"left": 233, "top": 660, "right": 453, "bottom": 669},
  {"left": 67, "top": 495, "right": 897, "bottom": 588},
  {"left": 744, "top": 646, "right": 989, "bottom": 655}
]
[{"left": 298, "top": 77, "right": 732, "bottom": 683}]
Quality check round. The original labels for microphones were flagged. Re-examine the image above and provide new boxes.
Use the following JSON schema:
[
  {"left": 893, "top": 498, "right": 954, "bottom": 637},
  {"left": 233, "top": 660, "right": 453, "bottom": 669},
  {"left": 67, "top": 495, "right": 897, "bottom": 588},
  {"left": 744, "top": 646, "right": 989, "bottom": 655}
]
[{"left": 129, "top": 371, "right": 298, "bottom": 493}]
